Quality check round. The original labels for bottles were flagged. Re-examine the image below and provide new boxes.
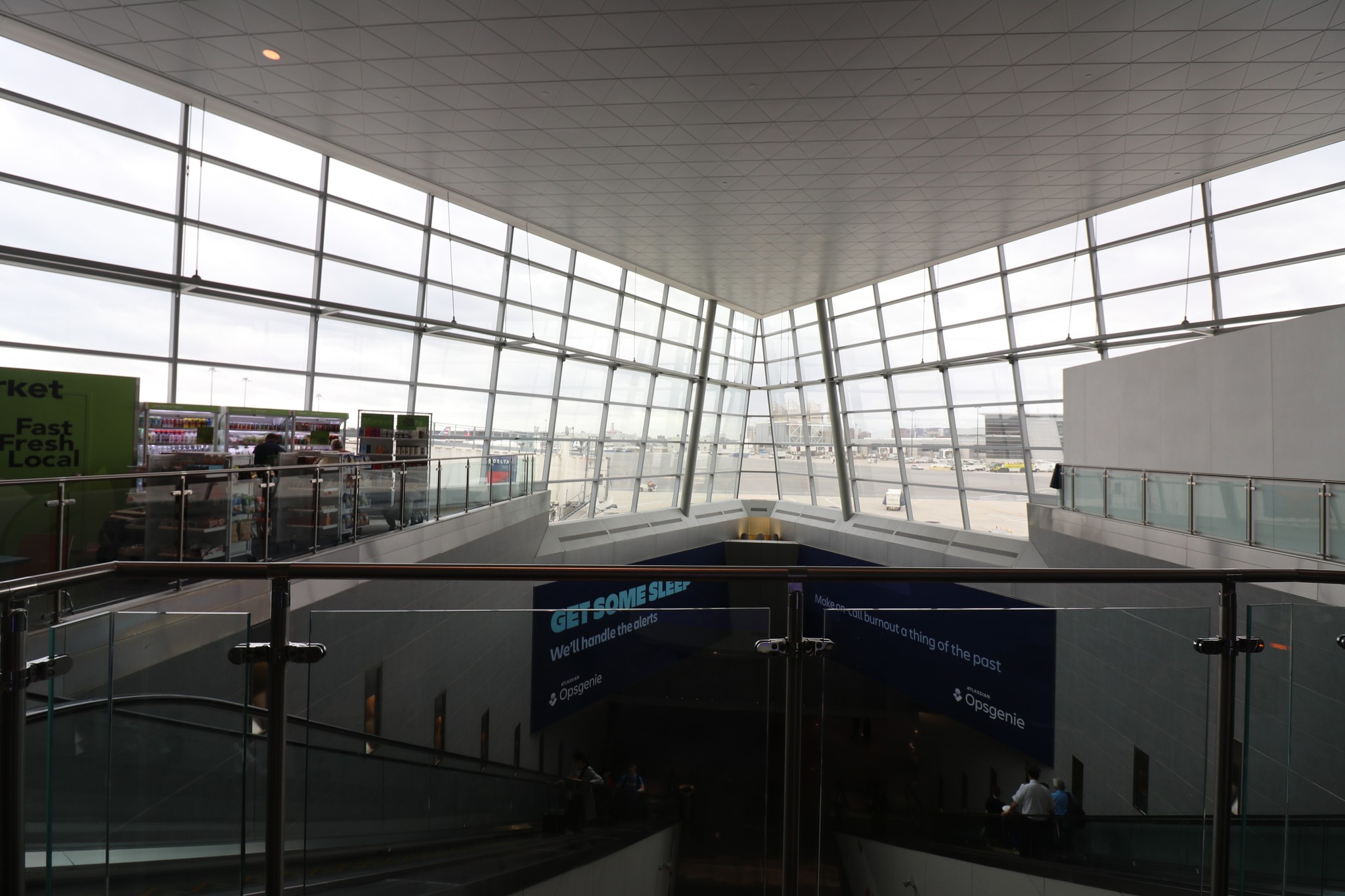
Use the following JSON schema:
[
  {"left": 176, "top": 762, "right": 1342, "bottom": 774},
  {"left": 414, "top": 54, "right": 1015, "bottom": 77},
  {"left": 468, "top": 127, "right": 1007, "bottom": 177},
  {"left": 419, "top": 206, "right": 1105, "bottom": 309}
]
[
  {"left": 382, "top": 429, "right": 392, "bottom": 437},
  {"left": 395, "top": 430, "right": 408, "bottom": 438},
  {"left": 207, "top": 418, "right": 211, "bottom": 427},
  {"left": 288, "top": 435, "right": 310, "bottom": 445},
  {"left": 329, "top": 433, "right": 339, "bottom": 445},
  {"left": 136, "top": 478, "right": 143, "bottom": 492},
  {"left": 296, "top": 422, "right": 340, "bottom": 432},
  {"left": 139, "top": 433, "right": 211, "bottom": 457},
  {"left": 396, "top": 445, "right": 420, "bottom": 455}
]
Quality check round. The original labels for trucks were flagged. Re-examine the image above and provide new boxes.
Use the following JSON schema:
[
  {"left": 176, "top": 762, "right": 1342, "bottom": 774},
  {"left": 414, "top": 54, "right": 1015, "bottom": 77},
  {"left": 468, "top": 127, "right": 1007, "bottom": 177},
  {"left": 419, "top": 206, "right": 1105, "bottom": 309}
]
[{"left": 768, "top": 450, "right": 786, "bottom": 459}]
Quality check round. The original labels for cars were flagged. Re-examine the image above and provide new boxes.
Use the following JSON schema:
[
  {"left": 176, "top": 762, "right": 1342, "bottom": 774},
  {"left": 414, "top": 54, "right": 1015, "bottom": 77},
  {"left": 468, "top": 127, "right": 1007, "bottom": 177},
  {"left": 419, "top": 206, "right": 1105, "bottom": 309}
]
[
  {"left": 889, "top": 452, "right": 1063, "bottom": 473},
  {"left": 911, "top": 464, "right": 924, "bottom": 470}
]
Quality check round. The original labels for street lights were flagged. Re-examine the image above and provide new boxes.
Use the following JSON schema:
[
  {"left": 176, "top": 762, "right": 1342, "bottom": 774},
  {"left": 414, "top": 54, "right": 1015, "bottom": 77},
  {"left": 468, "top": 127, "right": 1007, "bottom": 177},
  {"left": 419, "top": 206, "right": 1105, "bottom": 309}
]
[
  {"left": 316, "top": 393, "right": 322, "bottom": 412},
  {"left": 910, "top": 410, "right": 916, "bottom": 457},
  {"left": 242, "top": 377, "right": 248, "bottom": 407},
  {"left": 975, "top": 406, "right": 982, "bottom": 460},
  {"left": 544, "top": 419, "right": 548, "bottom": 438},
  {"left": 210, "top": 368, "right": 215, "bottom": 405}
]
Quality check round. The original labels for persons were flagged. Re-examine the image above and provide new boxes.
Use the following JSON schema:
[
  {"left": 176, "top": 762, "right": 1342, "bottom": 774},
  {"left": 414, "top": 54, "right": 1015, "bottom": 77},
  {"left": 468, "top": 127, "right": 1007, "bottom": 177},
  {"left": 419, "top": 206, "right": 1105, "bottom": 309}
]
[
  {"left": 253, "top": 434, "right": 286, "bottom": 475},
  {"left": 546, "top": 750, "right": 644, "bottom": 834},
  {"left": 985, "top": 765, "right": 1075, "bottom": 858},
  {"left": 331, "top": 438, "right": 352, "bottom": 453}
]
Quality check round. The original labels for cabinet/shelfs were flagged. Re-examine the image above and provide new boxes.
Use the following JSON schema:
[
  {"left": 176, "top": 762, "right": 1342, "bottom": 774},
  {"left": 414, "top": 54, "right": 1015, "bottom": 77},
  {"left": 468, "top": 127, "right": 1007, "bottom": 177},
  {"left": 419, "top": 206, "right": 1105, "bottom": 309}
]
[{"left": 106, "top": 409, "right": 431, "bottom": 567}]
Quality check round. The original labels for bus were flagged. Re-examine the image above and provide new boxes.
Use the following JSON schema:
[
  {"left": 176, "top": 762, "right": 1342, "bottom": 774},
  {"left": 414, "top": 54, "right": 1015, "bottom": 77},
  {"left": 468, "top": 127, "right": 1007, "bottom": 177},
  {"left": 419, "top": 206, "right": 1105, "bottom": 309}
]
[
  {"left": 875, "top": 447, "right": 890, "bottom": 460},
  {"left": 938, "top": 449, "right": 955, "bottom": 460}
]
